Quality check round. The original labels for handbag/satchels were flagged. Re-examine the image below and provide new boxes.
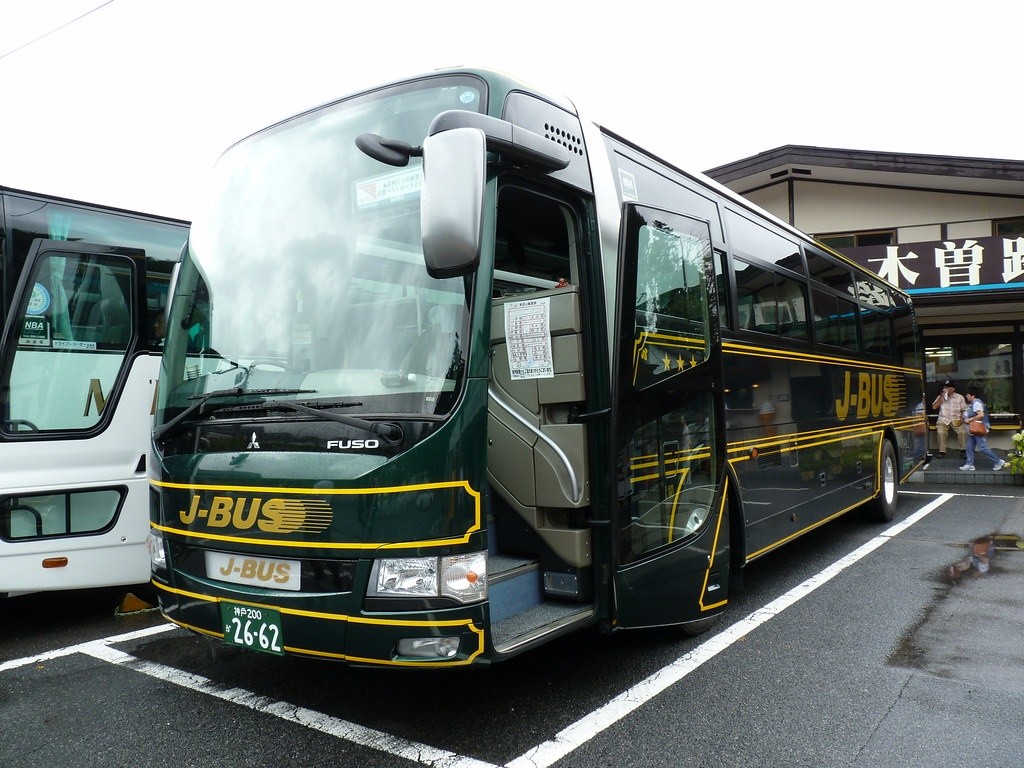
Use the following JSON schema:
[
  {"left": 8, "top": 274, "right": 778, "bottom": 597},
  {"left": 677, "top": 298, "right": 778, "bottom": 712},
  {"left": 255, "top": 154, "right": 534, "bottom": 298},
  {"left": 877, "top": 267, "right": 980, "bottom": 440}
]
[{"left": 970, "top": 415, "right": 989, "bottom": 436}]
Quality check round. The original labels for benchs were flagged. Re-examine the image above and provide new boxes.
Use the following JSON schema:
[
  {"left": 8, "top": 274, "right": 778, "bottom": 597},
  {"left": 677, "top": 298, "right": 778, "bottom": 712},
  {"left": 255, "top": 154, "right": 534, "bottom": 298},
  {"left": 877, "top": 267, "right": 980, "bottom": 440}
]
[{"left": 928, "top": 414, "right": 1022, "bottom": 455}]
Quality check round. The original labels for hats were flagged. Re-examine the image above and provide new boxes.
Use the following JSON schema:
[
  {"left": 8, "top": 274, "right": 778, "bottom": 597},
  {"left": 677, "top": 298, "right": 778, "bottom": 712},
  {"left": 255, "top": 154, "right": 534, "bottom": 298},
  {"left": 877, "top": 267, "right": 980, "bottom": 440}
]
[{"left": 943, "top": 380, "right": 956, "bottom": 388}]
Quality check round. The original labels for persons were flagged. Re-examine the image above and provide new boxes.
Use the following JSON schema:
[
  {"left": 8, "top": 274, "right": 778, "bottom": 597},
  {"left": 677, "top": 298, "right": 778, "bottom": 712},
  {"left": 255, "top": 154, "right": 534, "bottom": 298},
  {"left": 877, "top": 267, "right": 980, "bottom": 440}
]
[
  {"left": 946, "top": 537, "right": 995, "bottom": 578},
  {"left": 959, "top": 386, "right": 1005, "bottom": 471},
  {"left": 149, "top": 309, "right": 166, "bottom": 348},
  {"left": 914, "top": 402, "right": 926, "bottom": 453},
  {"left": 931, "top": 378, "right": 968, "bottom": 459},
  {"left": 680, "top": 415, "right": 692, "bottom": 486}
]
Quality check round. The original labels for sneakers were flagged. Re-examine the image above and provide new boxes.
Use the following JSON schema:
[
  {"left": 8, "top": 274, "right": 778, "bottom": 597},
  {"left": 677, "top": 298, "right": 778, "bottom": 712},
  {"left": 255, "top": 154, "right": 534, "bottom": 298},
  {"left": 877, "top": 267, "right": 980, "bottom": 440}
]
[
  {"left": 959, "top": 464, "right": 976, "bottom": 471},
  {"left": 992, "top": 459, "right": 1005, "bottom": 471}
]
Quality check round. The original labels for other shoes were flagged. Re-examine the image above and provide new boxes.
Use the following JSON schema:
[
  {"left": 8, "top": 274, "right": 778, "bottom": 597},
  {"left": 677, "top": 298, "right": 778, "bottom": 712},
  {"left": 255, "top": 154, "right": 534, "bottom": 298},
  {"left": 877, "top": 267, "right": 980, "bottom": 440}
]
[
  {"left": 937, "top": 452, "right": 944, "bottom": 457},
  {"left": 962, "top": 451, "right": 967, "bottom": 458}
]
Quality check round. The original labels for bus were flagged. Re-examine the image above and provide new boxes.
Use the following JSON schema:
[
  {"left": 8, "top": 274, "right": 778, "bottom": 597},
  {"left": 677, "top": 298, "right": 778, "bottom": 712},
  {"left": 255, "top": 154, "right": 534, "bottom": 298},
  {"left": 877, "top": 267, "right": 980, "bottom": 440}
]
[
  {"left": 148, "top": 69, "right": 933, "bottom": 671},
  {"left": 0, "top": 184, "right": 560, "bottom": 596}
]
